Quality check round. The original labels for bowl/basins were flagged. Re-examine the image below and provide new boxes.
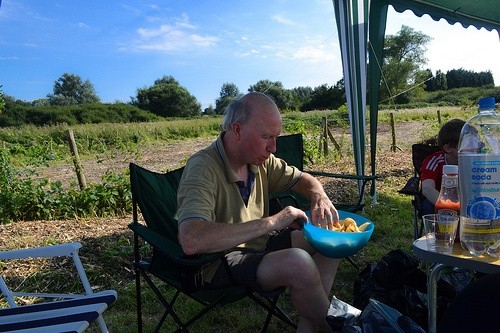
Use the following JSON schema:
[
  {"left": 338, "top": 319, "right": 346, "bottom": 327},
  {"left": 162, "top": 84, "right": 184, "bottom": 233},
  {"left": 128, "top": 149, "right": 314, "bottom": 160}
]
[{"left": 303, "top": 210, "right": 375, "bottom": 259}]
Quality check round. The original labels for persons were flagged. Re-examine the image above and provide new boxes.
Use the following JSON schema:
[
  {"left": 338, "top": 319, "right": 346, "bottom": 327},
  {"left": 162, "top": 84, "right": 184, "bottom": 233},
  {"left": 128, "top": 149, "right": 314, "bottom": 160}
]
[
  {"left": 417, "top": 118, "right": 480, "bottom": 234},
  {"left": 176, "top": 92, "right": 342, "bottom": 333}
]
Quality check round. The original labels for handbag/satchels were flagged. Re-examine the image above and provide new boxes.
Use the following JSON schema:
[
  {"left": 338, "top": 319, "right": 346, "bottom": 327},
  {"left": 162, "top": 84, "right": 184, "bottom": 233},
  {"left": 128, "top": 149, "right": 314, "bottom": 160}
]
[{"left": 326, "top": 248, "right": 499, "bottom": 333}]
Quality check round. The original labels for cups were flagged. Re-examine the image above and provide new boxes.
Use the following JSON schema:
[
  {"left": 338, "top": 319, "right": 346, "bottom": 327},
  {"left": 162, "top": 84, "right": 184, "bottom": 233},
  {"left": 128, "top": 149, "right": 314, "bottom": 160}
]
[{"left": 422, "top": 214, "right": 459, "bottom": 254}]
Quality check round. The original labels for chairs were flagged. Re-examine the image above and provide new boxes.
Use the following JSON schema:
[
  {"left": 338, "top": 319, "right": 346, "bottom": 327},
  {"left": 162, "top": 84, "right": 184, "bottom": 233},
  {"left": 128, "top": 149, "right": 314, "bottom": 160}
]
[
  {"left": 126, "top": 161, "right": 299, "bottom": 333},
  {"left": 260, "top": 133, "right": 380, "bottom": 275},
  {"left": 398, "top": 142, "right": 437, "bottom": 242},
  {"left": 0, "top": 243, "right": 122, "bottom": 333}
]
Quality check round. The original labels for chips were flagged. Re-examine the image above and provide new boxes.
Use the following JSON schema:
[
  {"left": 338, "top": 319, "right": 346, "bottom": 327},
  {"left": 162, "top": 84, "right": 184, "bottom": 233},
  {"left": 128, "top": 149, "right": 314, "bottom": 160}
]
[{"left": 319, "top": 217, "right": 371, "bottom": 232}]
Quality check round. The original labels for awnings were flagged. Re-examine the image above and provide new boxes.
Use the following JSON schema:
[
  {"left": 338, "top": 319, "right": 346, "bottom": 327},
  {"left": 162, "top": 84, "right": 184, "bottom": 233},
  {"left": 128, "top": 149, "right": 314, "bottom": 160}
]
[{"left": 368, "top": 0, "right": 500, "bottom": 205}]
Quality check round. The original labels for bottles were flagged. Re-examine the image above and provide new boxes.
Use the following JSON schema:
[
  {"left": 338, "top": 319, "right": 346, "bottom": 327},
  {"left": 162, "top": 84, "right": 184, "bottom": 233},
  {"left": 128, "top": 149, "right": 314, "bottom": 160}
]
[
  {"left": 434, "top": 165, "right": 461, "bottom": 241},
  {"left": 459, "top": 97, "right": 500, "bottom": 258}
]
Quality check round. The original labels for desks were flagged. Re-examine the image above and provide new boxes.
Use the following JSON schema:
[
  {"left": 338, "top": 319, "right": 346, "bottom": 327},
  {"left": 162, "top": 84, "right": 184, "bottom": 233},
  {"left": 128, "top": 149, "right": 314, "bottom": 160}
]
[{"left": 412, "top": 223, "right": 500, "bottom": 333}]
